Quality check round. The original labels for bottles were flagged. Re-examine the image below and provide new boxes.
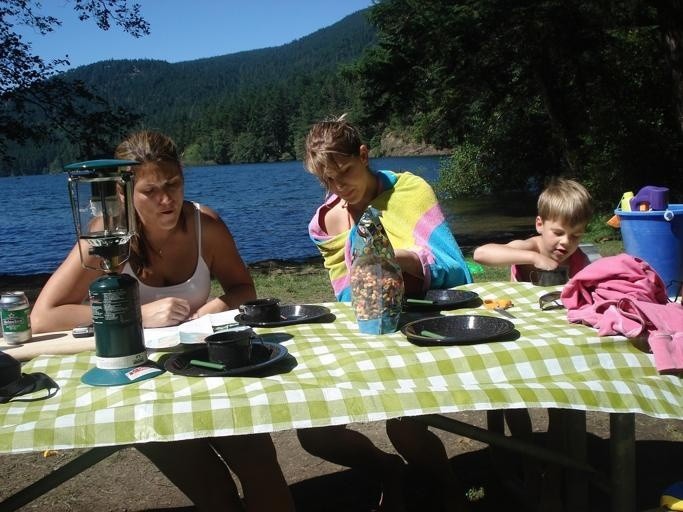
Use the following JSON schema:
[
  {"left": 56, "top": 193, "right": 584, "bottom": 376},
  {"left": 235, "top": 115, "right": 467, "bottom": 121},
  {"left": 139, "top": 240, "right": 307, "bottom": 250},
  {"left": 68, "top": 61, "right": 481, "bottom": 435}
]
[{"left": 621, "top": 191, "right": 635, "bottom": 211}]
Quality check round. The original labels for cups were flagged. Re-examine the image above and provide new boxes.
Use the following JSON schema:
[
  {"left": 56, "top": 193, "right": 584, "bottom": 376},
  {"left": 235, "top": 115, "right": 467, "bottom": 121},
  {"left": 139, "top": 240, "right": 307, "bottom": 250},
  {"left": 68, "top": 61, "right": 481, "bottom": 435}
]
[
  {"left": 239, "top": 297, "right": 283, "bottom": 322},
  {"left": 528, "top": 264, "right": 569, "bottom": 288},
  {"left": 203, "top": 330, "right": 265, "bottom": 369}
]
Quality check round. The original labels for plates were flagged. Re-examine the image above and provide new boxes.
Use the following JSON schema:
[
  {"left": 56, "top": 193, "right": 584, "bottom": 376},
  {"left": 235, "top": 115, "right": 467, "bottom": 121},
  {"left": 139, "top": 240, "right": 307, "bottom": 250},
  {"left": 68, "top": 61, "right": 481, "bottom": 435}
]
[
  {"left": 161, "top": 341, "right": 289, "bottom": 378},
  {"left": 234, "top": 304, "right": 332, "bottom": 329},
  {"left": 400, "top": 288, "right": 480, "bottom": 312},
  {"left": 397, "top": 313, "right": 517, "bottom": 346}
]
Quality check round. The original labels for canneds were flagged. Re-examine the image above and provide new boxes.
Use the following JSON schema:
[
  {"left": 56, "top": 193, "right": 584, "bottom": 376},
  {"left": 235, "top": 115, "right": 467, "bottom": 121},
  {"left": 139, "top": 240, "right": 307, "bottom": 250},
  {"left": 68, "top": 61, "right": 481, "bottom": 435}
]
[{"left": 0, "top": 291, "right": 33, "bottom": 345}]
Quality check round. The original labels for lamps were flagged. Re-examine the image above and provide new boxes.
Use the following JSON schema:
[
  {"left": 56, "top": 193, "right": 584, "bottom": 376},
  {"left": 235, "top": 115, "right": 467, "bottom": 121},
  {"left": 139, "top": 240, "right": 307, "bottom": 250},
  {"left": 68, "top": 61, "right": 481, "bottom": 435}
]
[{"left": 63, "top": 160, "right": 167, "bottom": 385}]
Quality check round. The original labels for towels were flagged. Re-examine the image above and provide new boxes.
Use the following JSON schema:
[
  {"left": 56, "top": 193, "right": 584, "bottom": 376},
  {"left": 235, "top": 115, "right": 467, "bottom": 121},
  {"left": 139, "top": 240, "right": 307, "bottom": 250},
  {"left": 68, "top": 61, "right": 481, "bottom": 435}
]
[{"left": 307, "top": 169, "right": 473, "bottom": 301}]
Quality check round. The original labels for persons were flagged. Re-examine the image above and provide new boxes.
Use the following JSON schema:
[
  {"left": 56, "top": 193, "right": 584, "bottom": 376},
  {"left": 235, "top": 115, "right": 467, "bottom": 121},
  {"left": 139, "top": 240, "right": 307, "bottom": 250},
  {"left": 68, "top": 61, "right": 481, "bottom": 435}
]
[
  {"left": 30, "top": 130, "right": 297, "bottom": 512},
  {"left": 474, "top": 175, "right": 594, "bottom": 439},
  {"left": 296, "top": 115, "right": 473, "bottom": 512}
]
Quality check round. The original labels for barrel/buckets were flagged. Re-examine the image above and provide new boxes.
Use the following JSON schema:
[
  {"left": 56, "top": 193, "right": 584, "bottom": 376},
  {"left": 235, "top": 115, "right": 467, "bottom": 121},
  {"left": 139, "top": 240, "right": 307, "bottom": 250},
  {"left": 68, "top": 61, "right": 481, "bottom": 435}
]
[{"left": 615, "top": 204, "right": 683, "bottom": 298}]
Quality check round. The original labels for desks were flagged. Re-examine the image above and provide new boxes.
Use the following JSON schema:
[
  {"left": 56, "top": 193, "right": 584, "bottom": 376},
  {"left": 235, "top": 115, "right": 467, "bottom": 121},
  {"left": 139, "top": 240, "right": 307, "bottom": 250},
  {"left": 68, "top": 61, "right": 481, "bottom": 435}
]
[{"left": 0, "top": 281, "right": 682, "bottom": 505}]
